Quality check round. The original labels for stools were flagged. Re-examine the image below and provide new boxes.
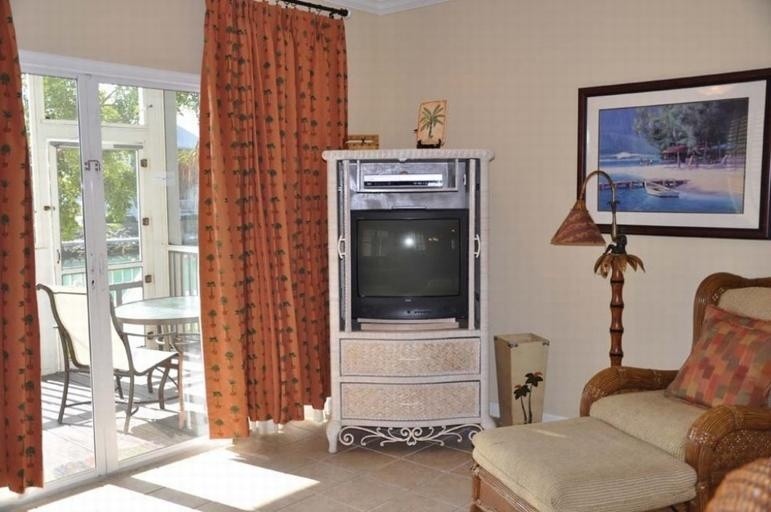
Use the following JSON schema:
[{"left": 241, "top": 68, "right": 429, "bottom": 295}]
[{"left": 468, "top": 414, "right": 698, "bottom": 512}]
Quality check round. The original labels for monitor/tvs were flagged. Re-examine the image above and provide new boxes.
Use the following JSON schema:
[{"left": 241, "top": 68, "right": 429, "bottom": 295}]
[{"left": 351, "top": 211, "right": 468, "bottom": 330}]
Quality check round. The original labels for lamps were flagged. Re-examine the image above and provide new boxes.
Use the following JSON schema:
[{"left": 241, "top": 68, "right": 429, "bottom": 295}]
[{"left": 550, "top": 200, "right": 607, "bottom": 247}]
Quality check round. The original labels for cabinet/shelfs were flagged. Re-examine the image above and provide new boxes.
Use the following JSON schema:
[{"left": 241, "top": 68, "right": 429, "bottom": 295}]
[{"left": 321, "top": 148, "right": 498, "bottom": 453}]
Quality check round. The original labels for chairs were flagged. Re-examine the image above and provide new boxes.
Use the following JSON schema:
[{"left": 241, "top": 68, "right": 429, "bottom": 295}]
[{"left": 36, "top": 283, "right": 180, "bottom": 434}]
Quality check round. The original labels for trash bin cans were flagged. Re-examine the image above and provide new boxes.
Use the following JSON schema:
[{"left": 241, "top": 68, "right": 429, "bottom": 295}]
[{"left": 494, "top": 333, "right": 549, "bottom": 425}]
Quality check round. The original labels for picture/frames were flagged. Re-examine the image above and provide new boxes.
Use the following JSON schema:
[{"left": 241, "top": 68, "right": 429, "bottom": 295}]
[{"left": 576, "top": 69, "right": 771, "bottom": 243}]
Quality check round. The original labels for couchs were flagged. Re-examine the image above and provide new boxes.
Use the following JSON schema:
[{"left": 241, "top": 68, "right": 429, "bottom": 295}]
[{"left": 580, "top": 271, "right": 771, "bottom": 512}]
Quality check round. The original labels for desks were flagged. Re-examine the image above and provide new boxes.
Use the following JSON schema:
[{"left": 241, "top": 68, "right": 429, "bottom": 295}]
[{"left": 114, "top": 295, "right": 200, "bottom": 393}]
